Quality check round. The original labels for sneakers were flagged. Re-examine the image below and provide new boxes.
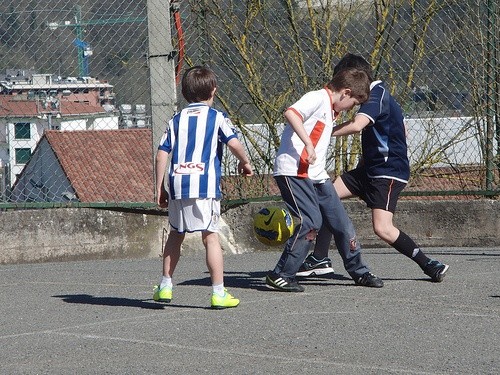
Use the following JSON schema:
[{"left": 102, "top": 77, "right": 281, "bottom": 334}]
[
  {"left": 211, "top": 291, "right": 239, "bottom": 308},
  {"left": 265, "top": 270, "right": 305, "bottom": 291},
  {"left": 153, "top": 286, "right": 172, "bottom": 302},
  {"left": 355, "top": 271, "right": 383, "bottom": 287},
  {"left": 424, "top": 260, "right": 449, "bottom": 282},
  {"left": 296, "top": 252, "right": 334, "bottom": 279}
]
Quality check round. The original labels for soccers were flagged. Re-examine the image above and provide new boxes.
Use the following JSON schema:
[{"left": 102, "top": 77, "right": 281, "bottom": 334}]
[{"left": 254, "top": 207, "right": 294, "bottom": 245}]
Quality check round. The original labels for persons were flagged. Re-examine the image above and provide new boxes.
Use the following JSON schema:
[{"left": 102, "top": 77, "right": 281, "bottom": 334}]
[
  {"left": 153, "top": 66, "right": 254, "bottom": 309},
  {"left": 295, "top": 52, "right": 450, "bottom": 283},
  {"left": 266, "top": 66, "right": 384, "bottom": 292}
]
